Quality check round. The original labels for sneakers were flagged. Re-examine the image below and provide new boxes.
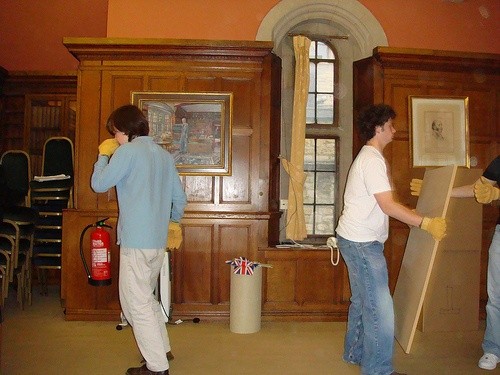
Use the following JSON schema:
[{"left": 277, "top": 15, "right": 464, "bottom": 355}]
[{"left": 479, "top": 353, "right": 500, "bottom": 370}]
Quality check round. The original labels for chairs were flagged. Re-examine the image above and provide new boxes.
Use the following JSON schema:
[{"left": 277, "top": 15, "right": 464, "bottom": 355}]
[{"left": 0, "top": 137, "right": 74, "bottom": 322}]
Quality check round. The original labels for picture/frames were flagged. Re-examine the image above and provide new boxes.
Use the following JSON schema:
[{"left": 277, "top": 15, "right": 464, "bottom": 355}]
[
  {"left": 130, "top": 91, "right": 234, "bottom": 176},
  {"left": 408, "top": 94, "right": 470, "bottom": 168}
]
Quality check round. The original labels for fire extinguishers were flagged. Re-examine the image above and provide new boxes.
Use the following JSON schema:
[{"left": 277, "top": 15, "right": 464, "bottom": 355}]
[{"left": 80, "top": 217, "right": 113, "bottom": 287}]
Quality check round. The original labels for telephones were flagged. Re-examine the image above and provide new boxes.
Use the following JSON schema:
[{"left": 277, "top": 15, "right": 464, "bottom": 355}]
[{"left": 327, "top": 237, "right": 338, "bottom": 248}]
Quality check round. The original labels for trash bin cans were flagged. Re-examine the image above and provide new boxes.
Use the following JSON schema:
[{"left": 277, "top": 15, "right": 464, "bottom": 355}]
[{"left": 230, "top": 263, "right": 262, "bottom": 334}]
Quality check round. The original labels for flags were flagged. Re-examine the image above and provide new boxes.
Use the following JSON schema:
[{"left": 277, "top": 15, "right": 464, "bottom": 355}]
[{"left": 225, "top": 257, "right": 260, "bottom": 275}]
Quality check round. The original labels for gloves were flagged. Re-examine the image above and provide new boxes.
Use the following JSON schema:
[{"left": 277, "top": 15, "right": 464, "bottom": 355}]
[
  {"left": 167, "top": 221, "right": 183, "bottom": 249},
  {"left": 421, "top": 217, "right": 447, "bottom": 241},
  {"left": 99, "top": 138, "right": 119, "bottom": 157},
  {"left": 474, "top": 176, "right": 500, "bottom": 204},
  {"left": 409, "top": 179, "right": 423, "bottom": 196}
]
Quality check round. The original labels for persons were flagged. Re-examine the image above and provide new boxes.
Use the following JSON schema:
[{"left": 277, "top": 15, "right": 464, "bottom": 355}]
[
  {"left": 410, "top": 154, "right": 500, "bottom": 370},
  {"left": 90, "top": 104, "right": 188, "bottom": 375},
  {"left": 335, "top": 104, "right": 447, "bottom": 375}
]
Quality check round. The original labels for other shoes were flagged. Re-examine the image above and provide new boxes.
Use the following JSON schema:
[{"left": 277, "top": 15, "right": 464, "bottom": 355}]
[
  {"left": 390, "top": 371, "right": 407, "bottom": 375},
  {"left": 126, "top": 360, "right": 170, "bottom": 375},
  {"left": 167, "top": 352, "right": 174, "bottom": 360}
]
